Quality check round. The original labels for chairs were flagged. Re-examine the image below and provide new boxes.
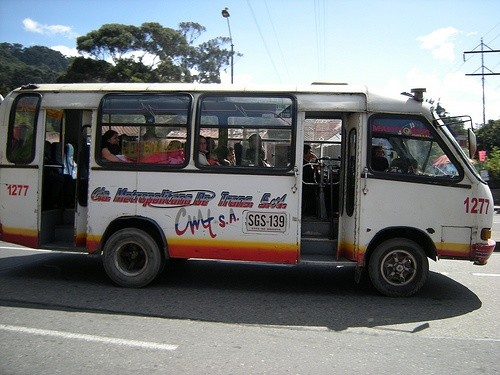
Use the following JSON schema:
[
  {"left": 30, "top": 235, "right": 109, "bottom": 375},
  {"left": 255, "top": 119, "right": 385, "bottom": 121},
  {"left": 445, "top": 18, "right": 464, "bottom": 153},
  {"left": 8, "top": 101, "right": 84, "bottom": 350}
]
[{"left": 44, "top": 139, "right": 79, "bottom": 201}]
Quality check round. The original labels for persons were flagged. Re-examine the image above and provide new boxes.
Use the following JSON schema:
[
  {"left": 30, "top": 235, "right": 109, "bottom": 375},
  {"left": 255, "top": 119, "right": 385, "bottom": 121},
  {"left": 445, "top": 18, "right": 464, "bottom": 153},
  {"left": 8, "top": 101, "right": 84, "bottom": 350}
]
[
  {"left": 371, "top": 145, "right": 389, "bottom": 172},
  {"left": 198, "top": 135, "right": 266, "bottom": 168},
  {"left": 302, "top": 144, "right": 318, "bottom": 169},
  {"left": 101, "top": 130, "right": 187, "bottom": 165}
]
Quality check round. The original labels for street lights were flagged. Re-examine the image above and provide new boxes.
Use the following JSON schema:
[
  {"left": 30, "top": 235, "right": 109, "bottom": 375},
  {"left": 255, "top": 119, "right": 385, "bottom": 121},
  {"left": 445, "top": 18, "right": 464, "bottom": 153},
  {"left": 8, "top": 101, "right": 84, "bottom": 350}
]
[{"left": 221, "top": 6, "right": 235, "bottom": 81}]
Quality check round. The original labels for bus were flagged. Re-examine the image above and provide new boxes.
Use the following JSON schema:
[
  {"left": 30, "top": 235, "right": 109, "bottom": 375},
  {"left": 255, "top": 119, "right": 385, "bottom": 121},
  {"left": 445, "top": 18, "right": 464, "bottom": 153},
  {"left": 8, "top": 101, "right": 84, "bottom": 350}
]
[{"left": 0, "top": 82, "right": 496, "bottom": 296}]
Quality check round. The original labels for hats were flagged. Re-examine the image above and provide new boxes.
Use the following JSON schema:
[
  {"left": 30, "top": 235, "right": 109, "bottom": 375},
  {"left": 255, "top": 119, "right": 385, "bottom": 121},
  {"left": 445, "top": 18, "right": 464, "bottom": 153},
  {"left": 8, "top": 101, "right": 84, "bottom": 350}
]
[{"left": 372, "top": 145, "right": 384, "bottom": 150}]
